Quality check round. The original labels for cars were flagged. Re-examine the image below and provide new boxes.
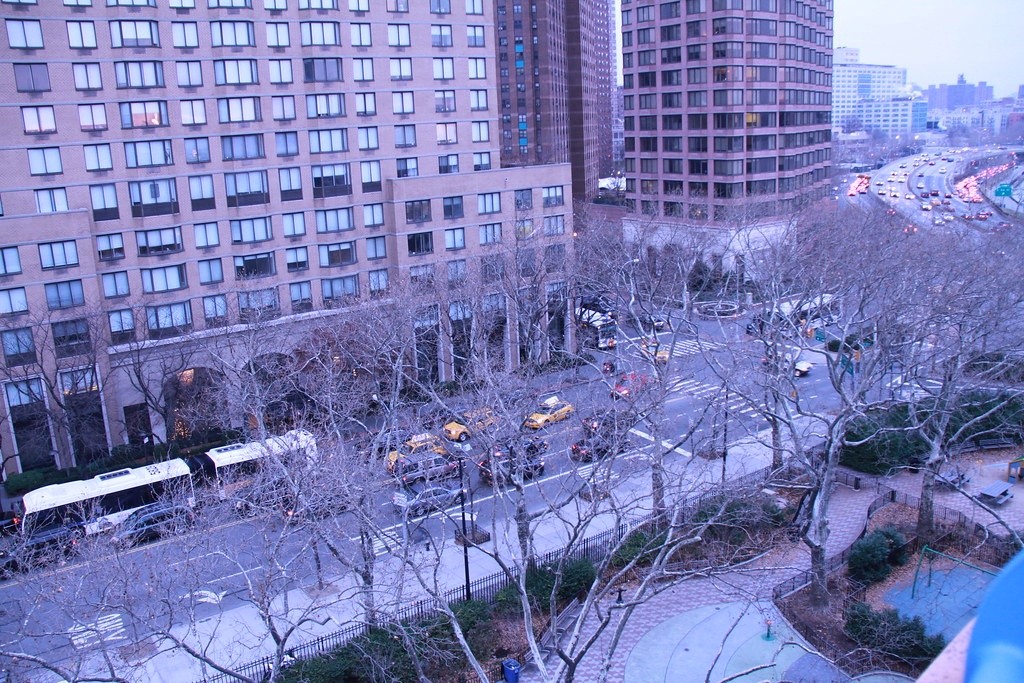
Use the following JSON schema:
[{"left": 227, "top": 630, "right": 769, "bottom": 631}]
[
  {"left": 761, "top": 351, "right": 814, "bottom": 378},
  {"left": 229, "top": 468, "right": 310, "bottom": 519},
  {"left": 624, "top": 311, "right": 664, "bottom": 333},
  {"left": 640, "top": 343, "right": 669, "bottom": 365},
  {"left": 524, "top": 396, "right": 575, "bottom": 429},
  {"left": 571, "top": 434, "right": 633, "bottom": 462},
  {"left": 278, "top": 480, "right": 368, "bottom": 525},
  {"left": 602, "top": 360, "right": 615, "bottom": 373},
  {"left": 581, "top": 407, "right": 638, "bottom": 438},
  {"left": 444, "top": 407, "right": 500, "bottom": 442},
  {"left": 351, "top": 426, "right": 415, "bottom": 463},
  {"left": 482, "top": 432, "right": 547, "bottom": 459},
  {"left": 477, "top": 454, "right": 545, "bottom": 490},
  {"left": 610, "top": 374, "right": 652, "bottom": 399},
  {"left": 838, "top": 135, "right": 1024, "bottom": 242}
]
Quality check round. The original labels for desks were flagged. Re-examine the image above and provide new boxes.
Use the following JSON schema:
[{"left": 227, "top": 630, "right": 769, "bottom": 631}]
[
  {"left": 982, "top": 479, "right": 1014, "bottom": 506},
  {"left": 935, "top": 470, "right": 965, "bottom": 491}
]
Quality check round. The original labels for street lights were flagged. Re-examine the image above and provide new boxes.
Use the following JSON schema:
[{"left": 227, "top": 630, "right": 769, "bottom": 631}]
[
  {"left": 452, "top": 443, "right": 474, "bottom": 600},
  {"left": 615, "top": 258, "right": 641, "bottom": 371}
]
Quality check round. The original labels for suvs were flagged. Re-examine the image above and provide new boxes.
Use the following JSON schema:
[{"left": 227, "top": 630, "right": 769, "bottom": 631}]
[
  {"left": 391, "top": 451, "right": 468, "bottom": 491},
  {"left": 105, "top": 498, "right": 200, "bottom": 553},
  {"left": 0, "top": 523, "right": 88, "bottom": 581},
  {"left": 385, "top": 431, "right": 447, "bottom": 477},
  {"left": 392, "top": 482, "right": 468, "bottom": 519}
]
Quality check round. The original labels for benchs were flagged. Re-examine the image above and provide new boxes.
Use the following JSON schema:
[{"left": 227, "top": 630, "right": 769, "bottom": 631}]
[
  {"left": 555, "top": 611, "right": 576, "bottom": 633},
  {"left": 638, "top": 565, "right": 663, "bottom": 584},
  {"left": 690, "top": 560, "right": 712, "bottom": 577},
  {"left": 917, "top": 448, "right": 943, "bottom": 466},
  {"left": 944, "top": 444, "right": 965, "bottom": 458},
  {"left": 539, "top": 626, "right": 562, "bottom": 653},
  {"left": 666, "top": 562, "right": 686, "bottom": 579},
  {"left": 566, "top": 597, "right": 585, "bottom": 617},
  {"left": 958, "top": 441, "right": 978, "bottom": 456},
  {"left": 979, "top": 439, "right": 1000, "bottom": 453},
  {"left": 998, "top": 492, "right": 1016, "bottom": 506},
  {"left": 954, "top": 476, "right": 972, "bottom": 489},
  {"left": 997, "top": 437, "right": 1017, "bottom": 452},
  {"left": 524, "top": 642, "right": 550, "bottom": 673}
]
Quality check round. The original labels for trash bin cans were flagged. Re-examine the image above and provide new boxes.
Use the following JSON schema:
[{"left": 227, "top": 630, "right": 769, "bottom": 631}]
[{"left": 501, "top": 658, "right": 521, "bottom": 683}]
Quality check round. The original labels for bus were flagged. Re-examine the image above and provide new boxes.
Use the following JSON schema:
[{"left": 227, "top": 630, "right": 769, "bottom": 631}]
[
  {"left": 15, "top": 458, "right": 196, "bottom": 561},
  {"left": 766, "top": 291, "right": 843, "bottom": 343},
  {"left": 562, "top": 306, "right": 620, "bottom": 349},
  {"left": 205, "top": 427, "right": 321, "bottom": 509}
]
